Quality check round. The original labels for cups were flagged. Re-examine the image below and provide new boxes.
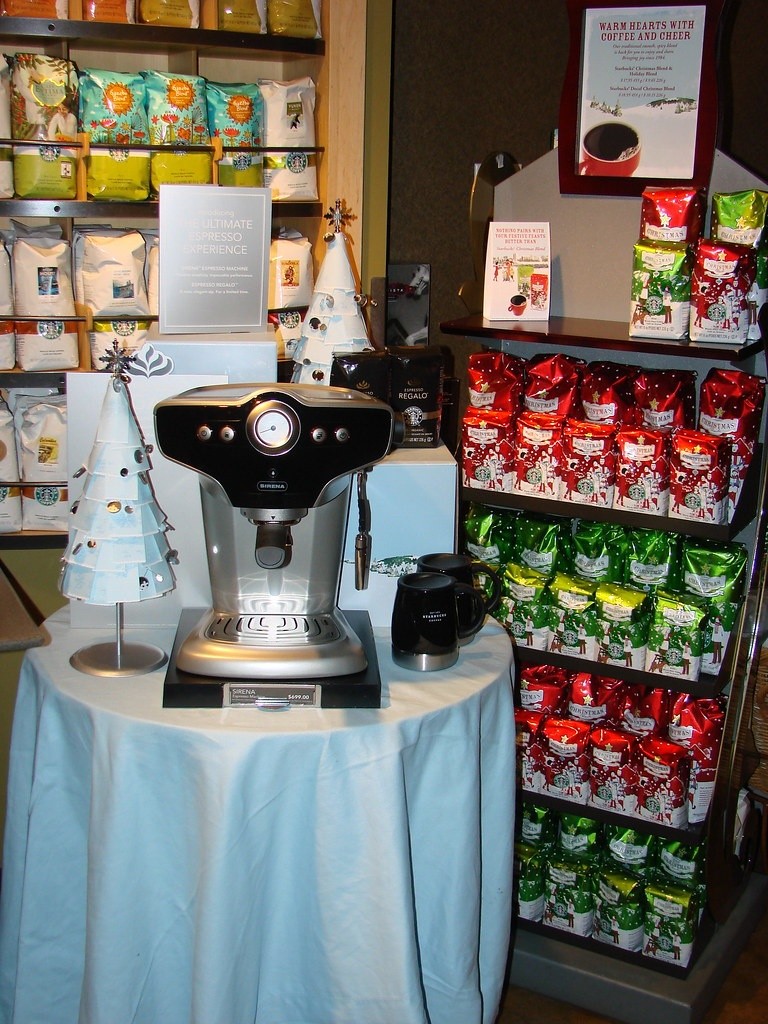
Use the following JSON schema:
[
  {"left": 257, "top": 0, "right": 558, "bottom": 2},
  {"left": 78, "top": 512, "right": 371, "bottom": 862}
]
[
  {"left": 391, "top": 574, "right": 485, "bottom": 672},
  {"left": 578, "top": 121, "right": 642, "bottom": 176},
  {"left": 414, "top": 552, "right": 501, "bottom": 648},
  {"left": 508, "top": 295, "right": 526, "bottom": 316}
]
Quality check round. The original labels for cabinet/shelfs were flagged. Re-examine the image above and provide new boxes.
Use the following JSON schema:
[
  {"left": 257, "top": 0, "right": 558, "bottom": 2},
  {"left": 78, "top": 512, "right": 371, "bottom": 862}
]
[
  {"left": 440, "top": 312, "right": 768, "bottom": 980},
  {"left": 0, "top": 16, "right": 324, "bottom": 550}
]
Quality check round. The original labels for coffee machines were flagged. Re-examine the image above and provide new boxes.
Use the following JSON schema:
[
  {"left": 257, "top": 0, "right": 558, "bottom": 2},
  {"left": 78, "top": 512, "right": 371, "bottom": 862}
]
[{"left": 153, "top": 382, "right": 405, "bottom": 708}]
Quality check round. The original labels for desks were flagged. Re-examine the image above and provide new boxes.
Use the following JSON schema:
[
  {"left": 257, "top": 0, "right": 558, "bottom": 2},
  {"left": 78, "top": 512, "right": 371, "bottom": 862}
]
[{"left": 0, "top": 563, "right": 515, "bottom": 1024}]
